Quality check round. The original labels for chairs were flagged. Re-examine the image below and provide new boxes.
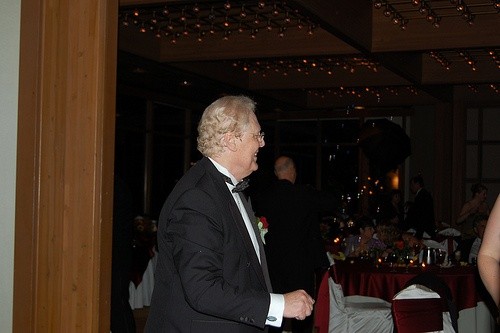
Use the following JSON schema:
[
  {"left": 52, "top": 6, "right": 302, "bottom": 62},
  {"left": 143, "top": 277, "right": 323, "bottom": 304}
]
[
  {"left": 314, "top": 251, "right": 394, "bottom": 333},
  {"left": 391, "top": 285, "right": 456, "bottom": 333}
]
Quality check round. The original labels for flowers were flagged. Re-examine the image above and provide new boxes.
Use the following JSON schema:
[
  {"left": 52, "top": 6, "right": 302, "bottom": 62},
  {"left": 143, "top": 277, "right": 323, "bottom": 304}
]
[{"left": 255, "top": 216, "right": 270, "bottom": 246}]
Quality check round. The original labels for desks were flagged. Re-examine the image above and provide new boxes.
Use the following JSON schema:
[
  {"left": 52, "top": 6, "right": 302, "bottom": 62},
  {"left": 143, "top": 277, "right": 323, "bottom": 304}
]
[{"left": 314, "top": 258, "right": 476, "bottom": 333}]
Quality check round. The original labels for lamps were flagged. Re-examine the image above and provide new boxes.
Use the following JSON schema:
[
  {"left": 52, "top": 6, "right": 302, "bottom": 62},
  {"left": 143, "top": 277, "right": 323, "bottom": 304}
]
[{"left": 119, "top": 0, "right": 500, "bottom": 116}]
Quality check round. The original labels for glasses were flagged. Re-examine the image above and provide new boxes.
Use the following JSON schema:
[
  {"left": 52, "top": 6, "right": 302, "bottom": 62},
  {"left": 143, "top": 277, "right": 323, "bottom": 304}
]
[{"left": 254, "top": 132, "right": 265, "bottom": 143}]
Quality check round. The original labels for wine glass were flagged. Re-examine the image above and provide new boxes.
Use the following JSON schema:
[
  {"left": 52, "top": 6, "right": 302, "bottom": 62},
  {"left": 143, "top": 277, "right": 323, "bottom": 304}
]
[{"left": 343, "top": 250, "right": 411, "bottom": 274}]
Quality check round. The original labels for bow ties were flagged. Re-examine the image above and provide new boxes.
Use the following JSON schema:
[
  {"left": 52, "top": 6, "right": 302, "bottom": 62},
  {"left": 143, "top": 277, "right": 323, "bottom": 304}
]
[{"left": 219, "top": 172, "right": 252, "bottom": 193}]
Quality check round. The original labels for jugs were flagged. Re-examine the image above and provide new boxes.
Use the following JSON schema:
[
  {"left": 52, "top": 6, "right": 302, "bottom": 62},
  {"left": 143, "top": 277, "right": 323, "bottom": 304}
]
[{"left": 420, "top": 246, "right": 436, "bottom": 265}]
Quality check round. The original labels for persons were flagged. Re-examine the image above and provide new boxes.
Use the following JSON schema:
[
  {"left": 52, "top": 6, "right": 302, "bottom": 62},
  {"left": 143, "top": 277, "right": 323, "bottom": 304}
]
[
  {"left": 144, "top": 96, "right": 315, "bottom": 333},
  {"left": 261, "top": 157, "right": 500, "bottom": 333}
]
